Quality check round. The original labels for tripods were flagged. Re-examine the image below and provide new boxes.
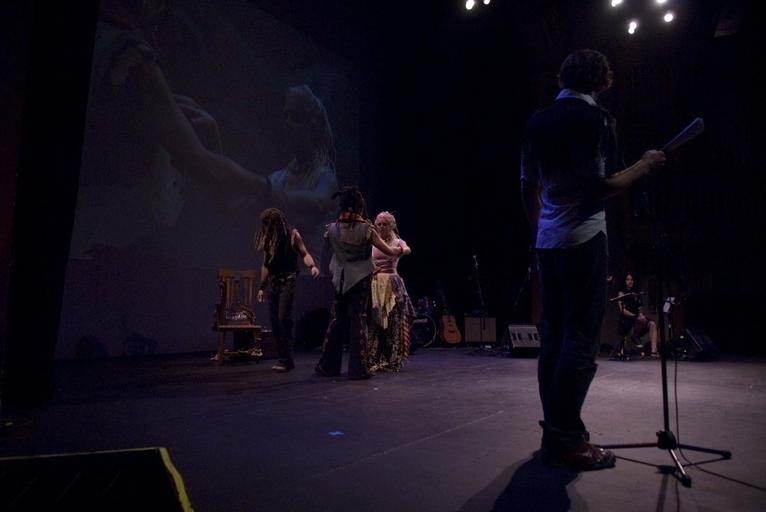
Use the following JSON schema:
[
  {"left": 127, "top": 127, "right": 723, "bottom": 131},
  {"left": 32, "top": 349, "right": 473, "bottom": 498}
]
[{"left": 595, "top": 159, "right": 731, "bottom": 486}]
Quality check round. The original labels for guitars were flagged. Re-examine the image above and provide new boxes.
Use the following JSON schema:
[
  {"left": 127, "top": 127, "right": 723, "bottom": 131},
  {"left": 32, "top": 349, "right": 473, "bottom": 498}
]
[{"left": 437, "top": 288, "right": 463, "bottom": 346}]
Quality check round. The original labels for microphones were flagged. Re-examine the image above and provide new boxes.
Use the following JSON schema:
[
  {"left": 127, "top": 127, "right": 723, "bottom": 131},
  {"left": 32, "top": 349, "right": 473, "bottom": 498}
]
[{"left": 473, "top": 255, "right": 478, "bottom": 266}]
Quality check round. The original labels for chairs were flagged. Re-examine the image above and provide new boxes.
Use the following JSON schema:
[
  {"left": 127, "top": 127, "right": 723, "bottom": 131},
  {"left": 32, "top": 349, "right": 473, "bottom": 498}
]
[{"left": 209, "top": 268, "right": 264, "bottom": 367}]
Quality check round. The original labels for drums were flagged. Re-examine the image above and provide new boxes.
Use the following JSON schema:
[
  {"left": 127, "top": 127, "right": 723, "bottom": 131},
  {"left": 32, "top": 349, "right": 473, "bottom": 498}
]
[
  {"left": 411, "top": 314, "right": 438, "bottom": 347},
  {"left": 417, "top": 299, "right": 436, "bottom": 312}
]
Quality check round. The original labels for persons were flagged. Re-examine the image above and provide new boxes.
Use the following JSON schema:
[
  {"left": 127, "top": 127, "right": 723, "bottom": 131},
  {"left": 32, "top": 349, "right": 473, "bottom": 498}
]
[
  {"left": 520, "top": 46, "right": 671, "bottom": 473},
  {"left": 254, "top": 207, "right": 321, "bottom": 374},
  {"left": 615, "top": 270, "right": 661, "bottom": 358},
  {"left": 311, "top": 181, "right": 408, "bottom": 380},
  {"left": 364, "top": 209, "right": 414, "bottom": 380},
  {"left": 172, "top": 80, "right": 342, "bottom": 224},
  {"left": 66, "top": 1, "right": 292, "bottom": 249}
]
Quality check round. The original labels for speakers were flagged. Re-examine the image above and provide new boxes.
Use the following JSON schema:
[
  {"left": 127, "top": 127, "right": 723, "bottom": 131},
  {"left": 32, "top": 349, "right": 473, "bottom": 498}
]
[
  {"left": 464, "top": 309, "right": 508, "bottom": 348},
  {"left": 501, "top": 324, "right": 542, "bottom": 358}
]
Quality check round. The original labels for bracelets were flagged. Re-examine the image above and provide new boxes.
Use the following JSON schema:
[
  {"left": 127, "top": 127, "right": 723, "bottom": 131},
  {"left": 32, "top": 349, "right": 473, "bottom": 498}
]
[
  {"left": 258, "top": 281, "right": 266, "bottom": 290},
  {"left": 309, "top": 265, "right": 316, "bottom": 271},
  {"left": 263, "top": 175, "right": 273, "bottom": 194}
]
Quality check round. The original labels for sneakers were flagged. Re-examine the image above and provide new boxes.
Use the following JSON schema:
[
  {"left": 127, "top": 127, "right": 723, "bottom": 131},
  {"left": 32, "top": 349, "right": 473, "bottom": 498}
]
[
  {"left": 539, "top": 435, "right": 617, "bottom": 469},
  {"left": 271, "top": 360, "right": 296, "bottom": 372}
]
